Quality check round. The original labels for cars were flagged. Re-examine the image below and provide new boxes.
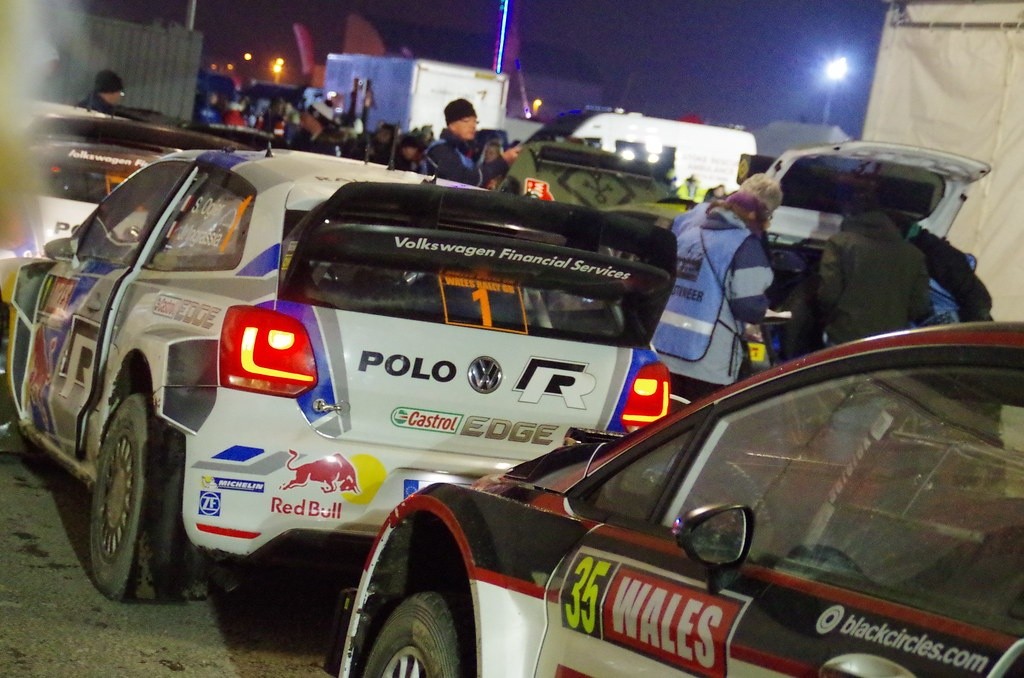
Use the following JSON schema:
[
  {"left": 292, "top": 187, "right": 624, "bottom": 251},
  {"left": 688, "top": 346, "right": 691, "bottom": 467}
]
[
  {"left": 0, "top": 135, "right": 681, "bottom": 605},
  {"left": 319, "top": 320, "right": 1024, "bottom": 678},
  {"left": 0, "top": 134, "right": 164, "bottom": 257}
]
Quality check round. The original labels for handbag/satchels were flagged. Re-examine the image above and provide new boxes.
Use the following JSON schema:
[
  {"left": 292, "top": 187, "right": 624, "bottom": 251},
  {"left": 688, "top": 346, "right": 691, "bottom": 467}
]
[{"left": 741, "top": 335, "right": 772, "bottom": 371}]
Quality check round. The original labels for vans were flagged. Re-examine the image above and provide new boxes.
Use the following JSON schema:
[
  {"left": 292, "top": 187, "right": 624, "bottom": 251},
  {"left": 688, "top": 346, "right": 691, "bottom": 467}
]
[{"left": 523, "top": 106, "right": 759, "bottom": 202}]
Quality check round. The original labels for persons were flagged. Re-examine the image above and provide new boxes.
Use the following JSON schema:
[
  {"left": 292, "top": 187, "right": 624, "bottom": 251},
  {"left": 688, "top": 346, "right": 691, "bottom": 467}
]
[
  {"left": 648, "top": 173, "right": 783, "bottom": 410},
  {"left": 885, "top": 205, "right": 1007, "bottom": 502},
  {"left": 197, "top": 84, "right": 522, "bottom": 188},
  {"left": 816, "top": 195, "right": 935, "bottom": 503},
  {"left": 663, "top": 169, "right": 728, "bottom": 209},
  {"left": 49, "top": 70, "right": 125, "bottom": 204}
]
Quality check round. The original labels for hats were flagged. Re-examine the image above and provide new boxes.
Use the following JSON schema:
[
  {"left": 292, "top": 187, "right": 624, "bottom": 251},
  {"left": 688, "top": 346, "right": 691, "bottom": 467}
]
[
  {"left": 445, "top": 99, "right": 475, "bottom": 126},
  {"left": 308, "top": 100, "right": 335, "bottom": 126},
  {"left": 728, "top": 192, "right": 766, "bottom": 235},
  {"left": 844, "top": 180, "right": 894, "bottom": 212},
  {"left": 94, "top": 71, "right": 123, "bottom": 93}
]
